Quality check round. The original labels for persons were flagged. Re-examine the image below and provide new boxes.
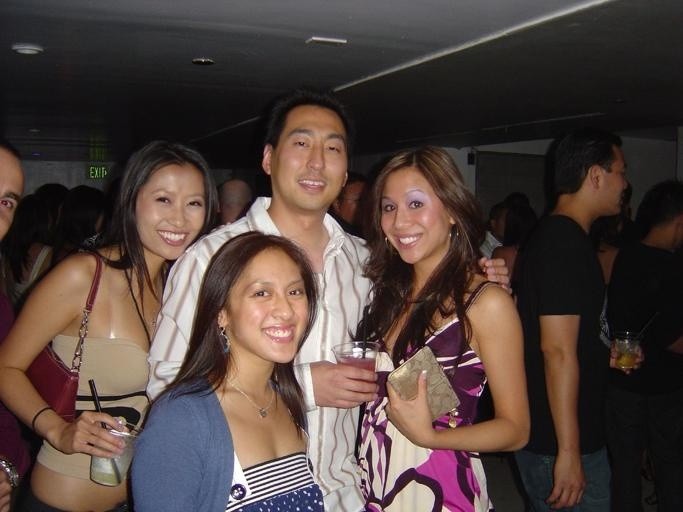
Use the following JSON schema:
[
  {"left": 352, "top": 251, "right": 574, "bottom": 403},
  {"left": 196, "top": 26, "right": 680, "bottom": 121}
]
[
  {"left": 512, "top": 125, "right": 643, "bottom": 512},
  {"left": 0, "top": 137, "right": 218, "bottom": 512},
  {"left": 357, "top": 143, "right": 532, "bottom": 512},
  {"left": 330, "top": 172, "right": 366, "bottom": 227},
  {"left": 591, "top": 181, "right": 633, "bottom": 285},
  {"left": 146, "top": 91, "right": 511, "bottom": 512},
  {"left": 1, "top": 144, "right": 35, "bottom": 510},
  {"left": 606, "top": 182, "right": 682, "bottom": 511},
  {"left": 477, "top": 192, "right": 536, "bottom": 278},
  {"left": 128, "top": 230, "right": 325, "bottom": 511},
  {"left": 215, "top": 178, "right": 251, "bottom": 223},
  {"left": 11, "top": 177, "right": 119, "bottom": 306}
]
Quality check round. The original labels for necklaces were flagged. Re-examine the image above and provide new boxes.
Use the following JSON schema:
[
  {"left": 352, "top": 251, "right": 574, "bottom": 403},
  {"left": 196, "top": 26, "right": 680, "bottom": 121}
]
[{"left": 226, "top": 378, "right": 276, "bottom": 420}]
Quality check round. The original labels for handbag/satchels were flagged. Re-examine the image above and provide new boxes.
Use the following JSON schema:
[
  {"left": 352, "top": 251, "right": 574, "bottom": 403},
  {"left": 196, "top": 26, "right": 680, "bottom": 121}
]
[{"left": 0, "top": 250, "right": 102, "bottom": 487}]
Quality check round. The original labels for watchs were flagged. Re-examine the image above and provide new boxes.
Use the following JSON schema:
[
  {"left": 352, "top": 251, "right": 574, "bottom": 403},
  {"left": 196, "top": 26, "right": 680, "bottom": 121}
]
[{"left": 0, "top": 457, "right": 22, "bottom": 489}]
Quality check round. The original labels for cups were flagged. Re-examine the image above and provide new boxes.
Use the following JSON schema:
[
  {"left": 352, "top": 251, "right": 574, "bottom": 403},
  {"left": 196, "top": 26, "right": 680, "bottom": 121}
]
[
  {"left": 612, "top": 332, "right": 642, "bottom": 369},
  {"left": 90, "top": 423, "right": 143, "bottom": 488},
  {"left": 331, "top": 342, "right": 379, "bottom": 376}
]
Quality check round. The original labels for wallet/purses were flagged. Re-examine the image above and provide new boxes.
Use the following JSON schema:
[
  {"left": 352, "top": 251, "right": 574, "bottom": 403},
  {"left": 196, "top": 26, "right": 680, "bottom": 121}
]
[{"left": 388, "top": 345, "right": 460, "bottom": 423}]
